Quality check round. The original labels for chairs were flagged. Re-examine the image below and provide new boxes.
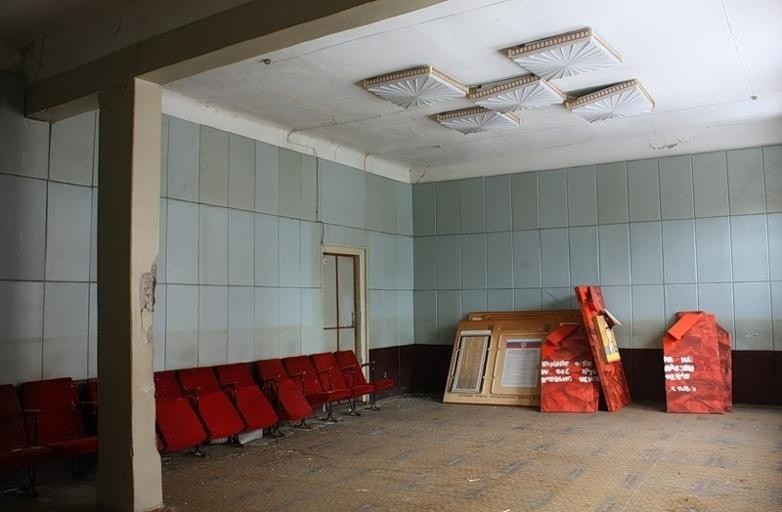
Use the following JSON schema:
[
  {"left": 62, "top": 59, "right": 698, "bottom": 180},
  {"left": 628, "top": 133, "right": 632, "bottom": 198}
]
[
  {"left": 87, "top": 358, "right": 313, "bottom": 478},
  {"left": 281, "top": 350, "right": 396, "bottom": 431},
  {"left": 2, "top": 378, "right": 100, "bottom": 498}
]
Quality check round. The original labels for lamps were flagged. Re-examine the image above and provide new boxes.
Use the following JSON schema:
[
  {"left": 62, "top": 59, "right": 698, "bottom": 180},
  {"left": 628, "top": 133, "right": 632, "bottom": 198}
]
[
  {"left": 468, "top": 74, "right": 566, "bottom": 116},
  {"left": 563, "top": 81, "right": 656, "bottom": 122},
  {"left": 357, "top": 65, "right": 470, "bottom": 110},
  {"left": 507, "top": 29, "right": 625, "bottom": 82},
  {"left": 436, "top": 106, "right": 522, "bottom": 134}
]
